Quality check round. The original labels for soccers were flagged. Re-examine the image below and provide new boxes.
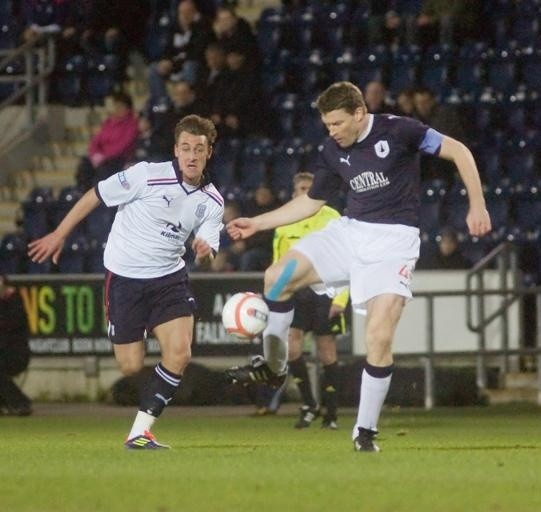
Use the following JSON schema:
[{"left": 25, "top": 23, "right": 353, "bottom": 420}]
[{"left": 222, "top": 289, "right": 269, "bottom": 340}]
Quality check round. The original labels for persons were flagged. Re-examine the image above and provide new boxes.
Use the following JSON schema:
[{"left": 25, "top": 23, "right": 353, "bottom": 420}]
[
  {"left": 23, "top": 0, "right": 472, "bottom": 271},
  {"left": 28, "top": 115, "right": 225, "bottom": 449},
  {"left": 1, "top": 271, "right": 31, "bottom": 416},
  {"left": 226, "top": 82, "right": 492, "bottom": 451},
  {"left": 272, "top": 172, "right": 349, "bottom": 429}
]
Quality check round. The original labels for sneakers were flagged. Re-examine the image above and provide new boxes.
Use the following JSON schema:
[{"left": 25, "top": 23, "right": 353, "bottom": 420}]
[
  {"left": 122, "top": 433, "right": 173, "bottom": 450},
  {"left": 249, "top": 400, "right": 339, "bottom": 430},
  {"left": 352, "top": 426, "right": 380, "bottom": 452},
  {"left": 225, "top": 359, "right": 289, "bottom": 392}
]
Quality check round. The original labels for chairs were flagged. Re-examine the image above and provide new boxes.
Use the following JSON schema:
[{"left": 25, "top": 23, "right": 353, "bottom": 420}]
[{"left": 1, "top": 0, "right": 539, "bottom": 291}]
